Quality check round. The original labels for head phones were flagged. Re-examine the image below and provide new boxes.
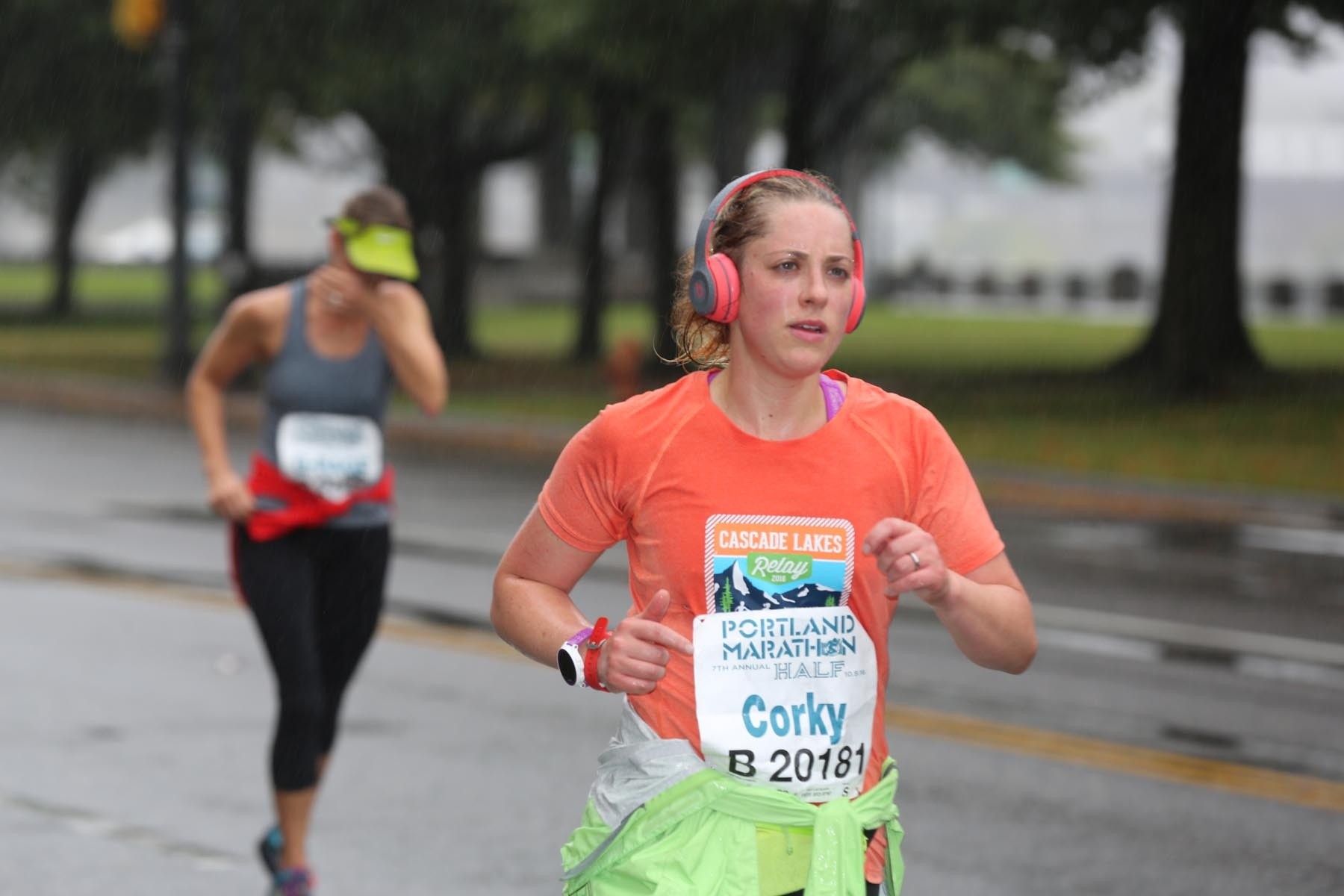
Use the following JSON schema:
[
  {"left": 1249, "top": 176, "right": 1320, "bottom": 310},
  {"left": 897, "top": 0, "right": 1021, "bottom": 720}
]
[{"left": 689, "top": 169, "right": 867, "bottom": 335}]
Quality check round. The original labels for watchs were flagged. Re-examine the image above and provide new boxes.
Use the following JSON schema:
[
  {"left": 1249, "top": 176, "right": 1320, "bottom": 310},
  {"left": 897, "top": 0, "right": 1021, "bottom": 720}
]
[{"left": 555, "top": 626, "right": 597, "bottom": 691}]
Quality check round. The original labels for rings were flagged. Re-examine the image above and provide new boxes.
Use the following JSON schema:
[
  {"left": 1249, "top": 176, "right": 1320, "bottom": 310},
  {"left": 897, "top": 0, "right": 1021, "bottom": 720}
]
[{"left": 910, "top": 552, "right": 921, "bottom": 571}]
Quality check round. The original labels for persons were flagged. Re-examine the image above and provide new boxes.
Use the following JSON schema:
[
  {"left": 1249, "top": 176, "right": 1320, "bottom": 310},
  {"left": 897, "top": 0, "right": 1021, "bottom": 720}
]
[
  {"left": 490, "top": 169, "right": 1037, "bottom": 895},
  {"left": 187, "top": 188, "right": 449, "bottom": 895}
]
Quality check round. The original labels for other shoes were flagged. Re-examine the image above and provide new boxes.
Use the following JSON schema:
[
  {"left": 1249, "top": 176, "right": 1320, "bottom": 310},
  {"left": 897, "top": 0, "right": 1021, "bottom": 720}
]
[{"left": 259, "top": 826, "right": 319, "bottom": 896}]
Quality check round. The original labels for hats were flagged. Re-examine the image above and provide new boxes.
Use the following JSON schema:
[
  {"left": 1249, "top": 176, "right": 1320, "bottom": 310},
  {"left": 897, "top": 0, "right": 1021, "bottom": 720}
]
[{"left": 333, "top": 215, "right": 419, "bottom": 280}]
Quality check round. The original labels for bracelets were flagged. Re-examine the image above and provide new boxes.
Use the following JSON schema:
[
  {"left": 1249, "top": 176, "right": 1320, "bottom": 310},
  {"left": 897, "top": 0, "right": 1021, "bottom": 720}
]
[{"left": 582, "top": 616, "right": 612, "bottom": 694}]
